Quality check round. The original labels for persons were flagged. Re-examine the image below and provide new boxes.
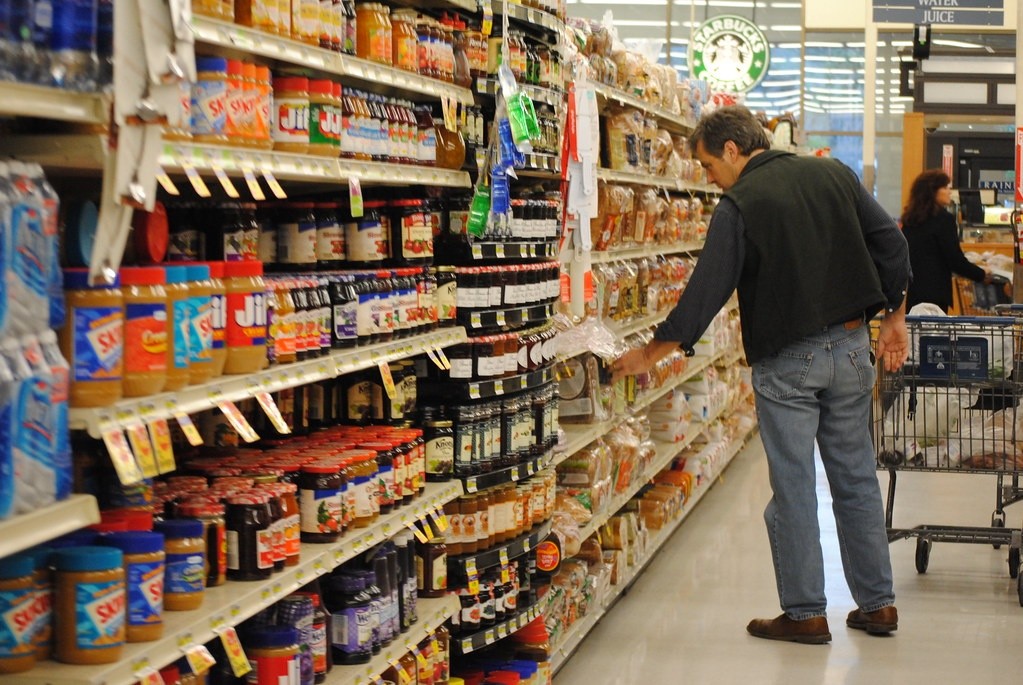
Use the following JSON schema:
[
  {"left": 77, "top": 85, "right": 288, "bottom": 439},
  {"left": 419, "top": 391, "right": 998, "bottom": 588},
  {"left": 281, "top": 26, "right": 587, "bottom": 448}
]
[
  {"left": 894, "top": 168, "right": 993, "bottom": 317},
  {"left": 604, "top": 104, "right": 910, "bottom": 646}
]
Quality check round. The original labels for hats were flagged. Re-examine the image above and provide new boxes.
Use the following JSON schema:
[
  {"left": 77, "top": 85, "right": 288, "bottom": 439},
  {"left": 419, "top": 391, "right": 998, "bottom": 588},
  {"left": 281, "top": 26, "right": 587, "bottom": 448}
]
[{"left": 963, "top": 387, "right": 1020, "bottom": 411}]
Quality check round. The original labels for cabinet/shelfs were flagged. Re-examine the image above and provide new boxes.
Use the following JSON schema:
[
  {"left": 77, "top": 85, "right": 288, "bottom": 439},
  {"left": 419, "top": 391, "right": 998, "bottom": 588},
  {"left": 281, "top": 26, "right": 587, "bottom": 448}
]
[{"left": 0, "top": 1, "right": 761, "bottom": 684}]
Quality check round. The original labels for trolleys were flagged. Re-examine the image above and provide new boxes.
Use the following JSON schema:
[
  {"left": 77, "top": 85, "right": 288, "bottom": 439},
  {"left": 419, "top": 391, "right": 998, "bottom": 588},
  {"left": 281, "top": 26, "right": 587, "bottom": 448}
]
[{"left": 868, "top": 273, "right": 1023, "bottom": 607}]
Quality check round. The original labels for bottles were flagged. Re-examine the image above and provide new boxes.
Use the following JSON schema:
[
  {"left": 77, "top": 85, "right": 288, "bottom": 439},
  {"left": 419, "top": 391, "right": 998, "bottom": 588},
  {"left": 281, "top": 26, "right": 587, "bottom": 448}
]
[
  {"left": 419, "top": 13, "right": 484, "bottom": 88},
  {"left": 1, "top": 163, "right": 64, "bottom": 335},
  {"left": 341, "top": 87, "right": 438, "bottom": 167},
  {"left": 193, "top": 0, "right": 418, "bottom": 71},
  {"left": 0, "top": 1, "right": 113, "bottom": 92},
  {"left": 1, "top": 330, "right": 71, "bottom": 519}
]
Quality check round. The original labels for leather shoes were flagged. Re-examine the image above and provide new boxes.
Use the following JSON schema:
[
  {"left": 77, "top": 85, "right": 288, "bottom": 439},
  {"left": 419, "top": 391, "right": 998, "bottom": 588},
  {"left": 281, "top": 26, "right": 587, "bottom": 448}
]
[
  {"left": 746, "top": 612, "right": 833, "bottom": 644},
  {"left": 846, "top": 606, "right": 899, "bottom": 633}
]
